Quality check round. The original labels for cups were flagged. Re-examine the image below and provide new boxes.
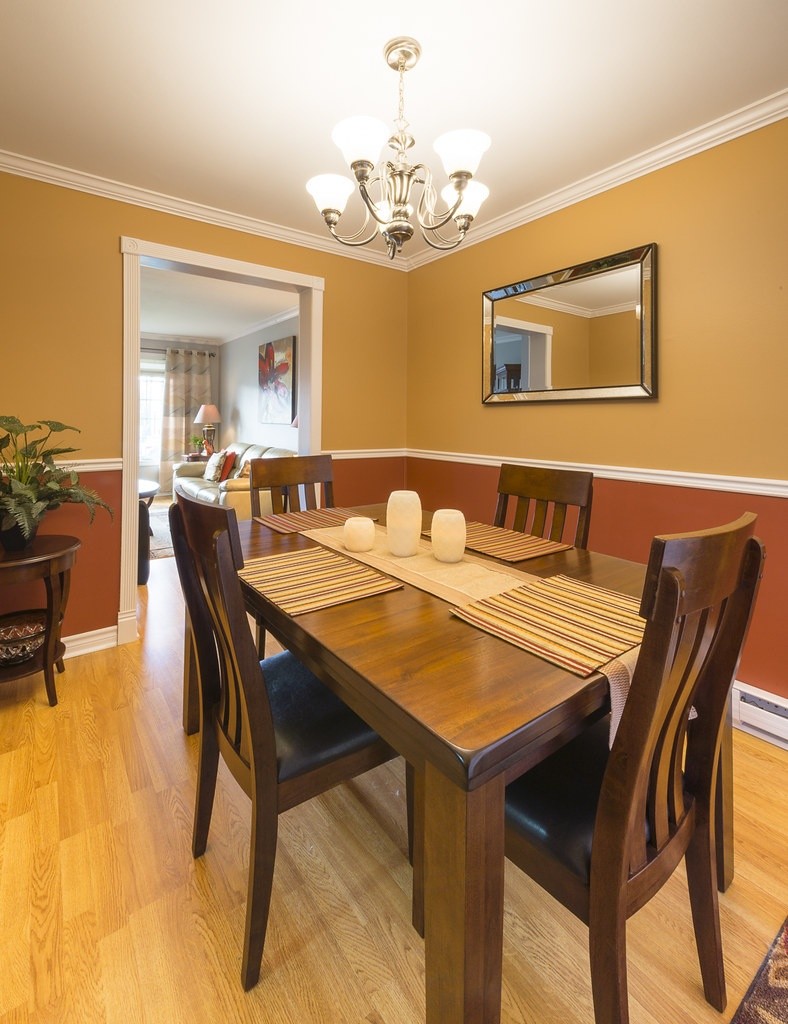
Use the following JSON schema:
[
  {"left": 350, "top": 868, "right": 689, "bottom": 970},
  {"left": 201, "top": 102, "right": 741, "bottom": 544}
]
[
  {"left": 385, "top": 490, "right": 422, "bottom": 557},
  {"left": 431, "top": 508, "right": 467, "bottom": 563},
  {"left": 342, "top": 517, "right": 376, "bottom": 553}
]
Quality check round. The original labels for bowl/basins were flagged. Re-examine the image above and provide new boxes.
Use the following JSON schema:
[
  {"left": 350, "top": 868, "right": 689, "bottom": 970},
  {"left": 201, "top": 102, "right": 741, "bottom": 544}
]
[{"left": 0, "top": 608, "right": 65, "bottom": 666}]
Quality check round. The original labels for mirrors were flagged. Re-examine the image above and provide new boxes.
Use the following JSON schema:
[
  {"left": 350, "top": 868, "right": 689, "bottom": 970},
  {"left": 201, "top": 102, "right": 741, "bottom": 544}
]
[{"left": 482, "top": 243, "right": 658, "bottom": 404}]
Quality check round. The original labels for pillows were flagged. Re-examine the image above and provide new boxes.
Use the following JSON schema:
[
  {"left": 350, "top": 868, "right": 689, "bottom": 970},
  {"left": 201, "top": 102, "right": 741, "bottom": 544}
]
[
  {"left": 234, "top": 461, "right": 252, "bottom": 479},
  {"left": 201, "top": 453, "right": 226, "bottom": 481},
  {"left": 219, "top": 449, "right": 236, "bottom": 482}
]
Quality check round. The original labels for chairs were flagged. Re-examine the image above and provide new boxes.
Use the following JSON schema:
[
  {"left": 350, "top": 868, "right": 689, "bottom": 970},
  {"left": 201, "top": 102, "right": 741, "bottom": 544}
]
[
  {"left": 169, "top": 485, "right": 415, "bottom": 993},
  {"left": 249, "top": 454, "right": 334, "bottom": 517},
  {"left": 493, "top": 463, "right": 594, "bottom": 551},
  {"left": 411, "top": 511, "right": 768, "bottom": 1024}
]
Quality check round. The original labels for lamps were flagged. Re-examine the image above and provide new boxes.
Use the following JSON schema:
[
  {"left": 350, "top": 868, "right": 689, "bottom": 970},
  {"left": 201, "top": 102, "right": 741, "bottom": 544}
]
[
  {"left": 193, "top": 405, "right": 222, "bottom": 449},
  {"left": 304, "top": 34, "right": 492, "bottom": 261}
]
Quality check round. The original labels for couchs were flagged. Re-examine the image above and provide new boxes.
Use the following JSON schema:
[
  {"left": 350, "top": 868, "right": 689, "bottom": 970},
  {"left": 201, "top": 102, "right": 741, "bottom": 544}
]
[{"left": 173, "top": 442, "right": 298, "bottom": 520}]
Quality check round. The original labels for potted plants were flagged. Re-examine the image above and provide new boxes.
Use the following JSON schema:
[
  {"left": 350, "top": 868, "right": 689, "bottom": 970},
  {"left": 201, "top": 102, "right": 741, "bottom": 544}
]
[
  {"left": 0, "top": 414, "right": 115, "bottom": 553},
  {"left": 192, "top": 436, "right": 204, "bottom": 455}
]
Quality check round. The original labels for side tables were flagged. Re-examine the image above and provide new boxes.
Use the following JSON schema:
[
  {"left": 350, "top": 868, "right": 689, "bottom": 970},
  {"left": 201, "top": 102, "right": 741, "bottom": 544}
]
[{"left": 181, "top": 455, "right": 213, "bottom": 462}]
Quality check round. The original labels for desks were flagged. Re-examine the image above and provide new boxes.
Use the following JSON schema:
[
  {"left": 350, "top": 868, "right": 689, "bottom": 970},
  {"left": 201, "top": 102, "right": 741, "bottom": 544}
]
[
  {"left": 0, "top": 535, "right": 81, "bottom": 708},
  {"left": 182, "top": 503, "right": 734, "bottom": 1024},
  {"left": 138, "top": 480, "right": 161, "bottom": 535}
]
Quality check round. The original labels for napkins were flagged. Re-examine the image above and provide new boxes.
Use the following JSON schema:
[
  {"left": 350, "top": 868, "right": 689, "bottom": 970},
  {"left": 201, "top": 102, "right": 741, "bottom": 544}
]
[
  {"left": 422, "top": 520, "right": 575, "bottom": 562},
  {"left": 253, "top": 507, "right": 378, "bottom": 533},
  {"left": 448, "top": 573, "right": 648, "bottom": 678},
  {"left": 237, "top": 546, "right": 404, "bottom": 617}
]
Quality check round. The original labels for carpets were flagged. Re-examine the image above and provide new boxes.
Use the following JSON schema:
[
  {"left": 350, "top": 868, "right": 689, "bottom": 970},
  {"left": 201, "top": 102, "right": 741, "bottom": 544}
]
[{"left": 730, "top": 916, "right": 788, "bottom": 1024}]
